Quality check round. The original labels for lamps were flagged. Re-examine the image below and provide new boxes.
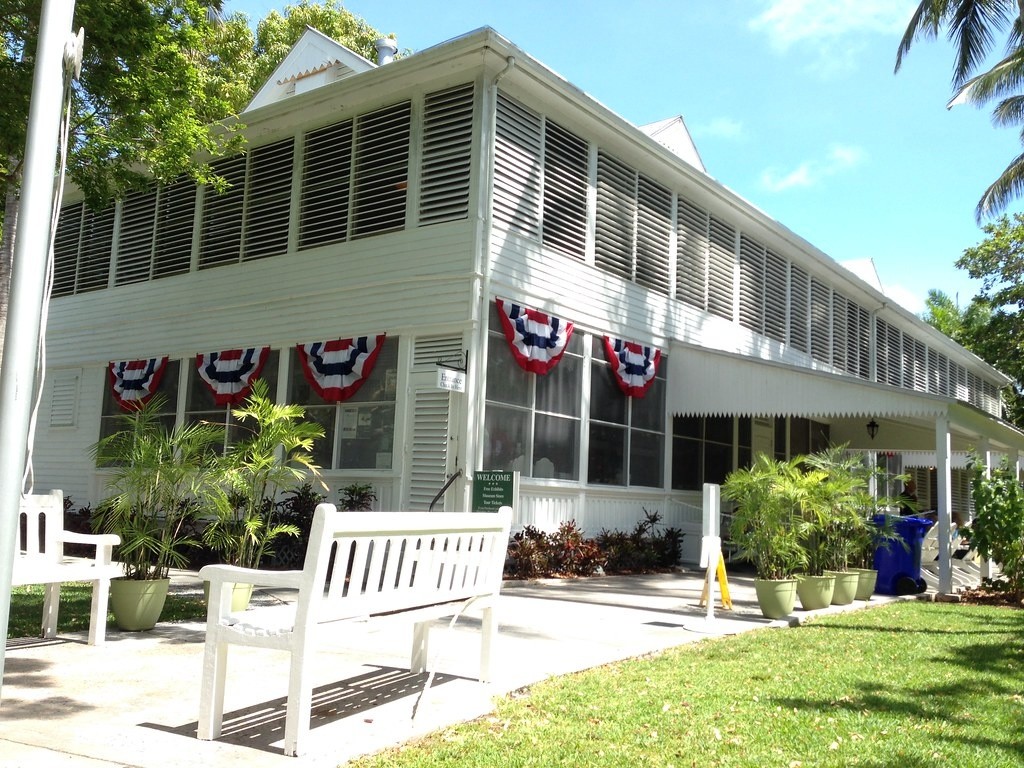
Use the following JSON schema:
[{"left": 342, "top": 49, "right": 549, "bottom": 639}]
[{"left": 866, "top": 418, "right": 879, "bottom": 441}]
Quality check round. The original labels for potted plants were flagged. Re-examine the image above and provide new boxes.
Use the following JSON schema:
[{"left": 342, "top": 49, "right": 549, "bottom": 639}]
[
  {"left": 718, "top": 439, "right": 922, "bottom": 620},
  {"left": 84, "top": 377, "right": 329, "bottom": 632}
]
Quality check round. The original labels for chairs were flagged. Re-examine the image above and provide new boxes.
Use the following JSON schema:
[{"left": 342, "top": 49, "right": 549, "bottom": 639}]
[{"left": 922, "top": 521, "right": 981, "bottom": 593}]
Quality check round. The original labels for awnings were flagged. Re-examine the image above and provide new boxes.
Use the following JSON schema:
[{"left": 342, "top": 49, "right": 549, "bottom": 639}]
[{"left": 669, "top": 338, "right": 1024, "bottom": 453}]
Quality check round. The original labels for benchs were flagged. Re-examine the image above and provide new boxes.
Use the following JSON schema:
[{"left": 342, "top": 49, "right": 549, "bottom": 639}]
[
  {"left": 10, "top": 489, "right": 121, "bottom": 646},
  {"left": 197, "top": 502, "right": 515, "bottom": 759}
]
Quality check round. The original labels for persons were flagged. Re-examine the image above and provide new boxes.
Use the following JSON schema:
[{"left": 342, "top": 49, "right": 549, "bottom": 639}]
[
  {"left": 934, "top": 518, "right": 978, "bottom": 561},
  {"left": 950, "top": 512, "right": 962, "bottom": 541},
  {"left": 898, "top": 480, "right": 919, "bottom": 517}
]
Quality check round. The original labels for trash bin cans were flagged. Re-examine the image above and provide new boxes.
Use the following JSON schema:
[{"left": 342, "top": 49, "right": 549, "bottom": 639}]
[{"left": 870, "top": 513, "right": 934, "bottom": 597}]
